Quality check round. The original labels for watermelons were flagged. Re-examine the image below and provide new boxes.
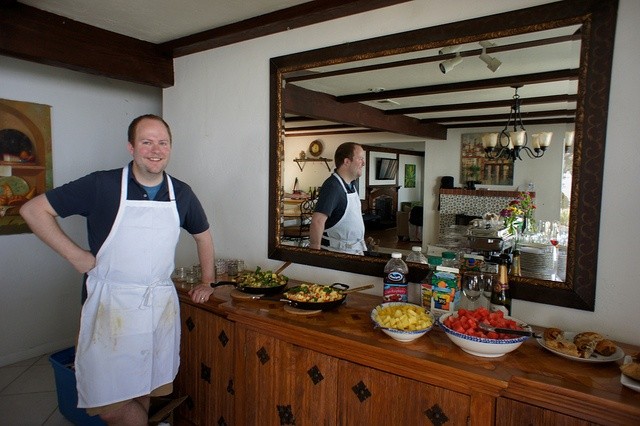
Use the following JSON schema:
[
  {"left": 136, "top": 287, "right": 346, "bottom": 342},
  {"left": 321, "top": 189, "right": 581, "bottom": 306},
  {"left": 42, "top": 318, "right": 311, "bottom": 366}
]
[{"left": 443, "top": 307, "right": 526, "bottom": 338}]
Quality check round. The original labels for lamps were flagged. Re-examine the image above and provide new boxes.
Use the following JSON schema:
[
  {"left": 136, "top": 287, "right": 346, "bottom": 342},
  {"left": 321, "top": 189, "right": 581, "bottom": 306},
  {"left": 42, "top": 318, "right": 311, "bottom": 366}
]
[{"left": 481, "top": 85, "right": 554, "bottom": 160}]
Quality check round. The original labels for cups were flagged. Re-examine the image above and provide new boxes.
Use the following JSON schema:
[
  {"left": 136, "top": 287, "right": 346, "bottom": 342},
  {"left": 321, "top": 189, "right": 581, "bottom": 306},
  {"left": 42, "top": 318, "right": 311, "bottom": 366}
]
[
  {"left": 193, "top": 266, "right": 201, "bottom": 280},
  {"left": 216, "top": 259, "right": 227, "bottom": 274},
  {"left": 231, "top": 260, "right": 244, "bottom": 276},
  {"left": 175, "top": 268, "right": 184, "bottom": 278},
  {"left": 186, "top": 273, "right": 195, "bottom": 283}
]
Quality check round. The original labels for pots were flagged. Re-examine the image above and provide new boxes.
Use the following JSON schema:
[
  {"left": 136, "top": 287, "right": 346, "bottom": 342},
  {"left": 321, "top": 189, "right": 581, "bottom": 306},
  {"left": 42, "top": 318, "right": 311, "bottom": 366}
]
[
  {"left": 280, "top": 283, "right": 348, "bottom": 308},
  {"left": 211, "top": 272, "right": 288, "bottom": 293}
]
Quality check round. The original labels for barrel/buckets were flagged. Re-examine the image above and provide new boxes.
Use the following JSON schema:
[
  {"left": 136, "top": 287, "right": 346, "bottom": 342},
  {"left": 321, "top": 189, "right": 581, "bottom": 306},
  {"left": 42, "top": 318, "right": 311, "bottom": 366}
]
[{"left": 49, "top": 345, "right": 100, "bottom": 426}]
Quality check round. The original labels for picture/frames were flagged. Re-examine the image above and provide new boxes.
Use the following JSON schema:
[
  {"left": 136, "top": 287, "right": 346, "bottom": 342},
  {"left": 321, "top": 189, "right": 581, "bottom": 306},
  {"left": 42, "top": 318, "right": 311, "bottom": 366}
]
[{"left": 404, "top": 164, "right": 416, "bottom": 188}]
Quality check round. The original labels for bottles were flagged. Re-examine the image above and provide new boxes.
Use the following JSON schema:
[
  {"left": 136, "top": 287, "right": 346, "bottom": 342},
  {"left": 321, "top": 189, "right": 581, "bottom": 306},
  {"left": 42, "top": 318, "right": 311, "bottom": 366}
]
[
  {"left": 384, "top": 252, "right": 408, "bottom": 303},
  {"left": 492, "top": 254, "right": 511, "bottom": 321},
  {"left": 443, "top": 252, "right": 455, "bottom": 269},
  {"left": 510, "top": 251, "right": 521, "bottom": 277},
  {"left": 406, "top": 246, "right": 427, "bottom": 263},
  {"left": 422, "top": 256, "right": 441, "bottom": 307}
]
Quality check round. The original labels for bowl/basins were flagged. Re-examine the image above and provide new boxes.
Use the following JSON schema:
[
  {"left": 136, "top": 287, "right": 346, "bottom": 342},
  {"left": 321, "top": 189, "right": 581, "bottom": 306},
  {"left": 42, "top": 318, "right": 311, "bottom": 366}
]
[
  {"left": 440, "top": 312, "right": 532, "bottom": 358},
  {"left": 371, "top": 302, "right": 436, "bottom": 341}
]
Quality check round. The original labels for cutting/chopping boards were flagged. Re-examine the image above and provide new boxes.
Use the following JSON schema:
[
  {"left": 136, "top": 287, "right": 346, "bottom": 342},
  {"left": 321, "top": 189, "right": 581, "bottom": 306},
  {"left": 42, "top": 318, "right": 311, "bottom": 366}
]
[
  {"left": 283, "top": 304, "right": 322, "bottom": 315},
  {"left": 231, "top": 289, "right": 264, "bottom": 299}
]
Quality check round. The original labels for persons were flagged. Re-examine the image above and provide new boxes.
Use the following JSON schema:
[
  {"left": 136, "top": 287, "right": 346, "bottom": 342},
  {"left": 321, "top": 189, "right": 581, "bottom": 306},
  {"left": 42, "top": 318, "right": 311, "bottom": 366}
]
[
  {"left": 310, "top": 142, "right": 372, "bottom": 255},
  {"left": 19, "top": 114, "right": 215, "bottom": 426}
]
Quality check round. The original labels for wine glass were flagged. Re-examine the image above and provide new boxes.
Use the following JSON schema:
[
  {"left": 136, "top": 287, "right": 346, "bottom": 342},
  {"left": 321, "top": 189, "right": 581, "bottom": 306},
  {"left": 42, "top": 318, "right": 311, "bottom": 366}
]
[
  {"left": 481, "top": 273, "right": 492, "bottom": 310},
  {"left": 462, "top": 271, "right": 484, "bottom": 312}
]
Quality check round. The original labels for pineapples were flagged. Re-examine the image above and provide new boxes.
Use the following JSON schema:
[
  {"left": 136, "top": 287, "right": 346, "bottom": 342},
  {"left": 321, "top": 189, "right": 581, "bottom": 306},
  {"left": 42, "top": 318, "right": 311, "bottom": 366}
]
[{"left": 376, "top": 303, "right": 433, "bottom": 332}]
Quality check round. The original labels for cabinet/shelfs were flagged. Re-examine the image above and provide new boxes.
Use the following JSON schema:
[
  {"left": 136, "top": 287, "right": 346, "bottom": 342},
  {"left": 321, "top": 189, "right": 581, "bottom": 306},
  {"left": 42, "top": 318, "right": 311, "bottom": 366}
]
[
  {"left": 369, "top": 185, "right": 400, "bottom": 216},
  {"left": 495, "top": 330, "right": 640, "bottom": 425},
  {"left": 174, "top": 270, "right": 234, "bottom": 425},
  {"left": 236, "top": 284, "right": 494, "bottom": 425}
]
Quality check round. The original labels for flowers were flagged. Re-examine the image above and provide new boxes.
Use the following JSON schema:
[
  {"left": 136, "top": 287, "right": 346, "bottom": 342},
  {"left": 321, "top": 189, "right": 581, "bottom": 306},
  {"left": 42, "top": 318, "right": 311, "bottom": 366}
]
[
  {"left": 500, "top": 200, "right": 523, "bottom": 235},
  {"left": 519, "top": 191, "right": 537, "bottom": 217}
]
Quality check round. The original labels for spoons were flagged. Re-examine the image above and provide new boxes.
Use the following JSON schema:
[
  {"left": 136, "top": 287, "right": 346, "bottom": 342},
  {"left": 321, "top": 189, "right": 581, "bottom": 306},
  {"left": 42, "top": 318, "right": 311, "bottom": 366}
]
[{"left": 479, "top": 322, "right": 540, "bottom": 339}]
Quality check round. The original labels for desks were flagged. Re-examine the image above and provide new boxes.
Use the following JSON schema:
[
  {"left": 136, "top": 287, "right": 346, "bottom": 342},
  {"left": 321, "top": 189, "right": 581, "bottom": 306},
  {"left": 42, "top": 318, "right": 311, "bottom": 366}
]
[{"left": 439, "top": 189, "right": 536, "bottom": 235}]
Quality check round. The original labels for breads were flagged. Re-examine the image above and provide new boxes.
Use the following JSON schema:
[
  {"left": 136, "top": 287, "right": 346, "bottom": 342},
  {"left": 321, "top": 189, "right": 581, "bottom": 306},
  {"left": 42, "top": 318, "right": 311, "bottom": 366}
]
[
  {"left": 596, "top": 338, "right": 617, "bottom": 355},
  {"left": 573, "top": 332, "right": 604, "bottom": 359},
  {"left": 544, "top": 327, "right": 582, "bottom": 357}
]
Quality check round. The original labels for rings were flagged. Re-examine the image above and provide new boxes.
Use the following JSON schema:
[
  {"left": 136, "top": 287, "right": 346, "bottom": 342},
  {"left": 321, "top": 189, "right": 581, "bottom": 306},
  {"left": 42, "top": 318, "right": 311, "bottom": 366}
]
[{"left": 200, "top": 297, "right": 205, "bottom": 300}]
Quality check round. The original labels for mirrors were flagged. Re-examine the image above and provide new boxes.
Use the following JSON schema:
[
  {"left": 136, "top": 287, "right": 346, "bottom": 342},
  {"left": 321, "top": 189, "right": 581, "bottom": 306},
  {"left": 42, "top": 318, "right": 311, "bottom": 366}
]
[{"left": 267, "top": 0, "right": 619, "bottom": 312}]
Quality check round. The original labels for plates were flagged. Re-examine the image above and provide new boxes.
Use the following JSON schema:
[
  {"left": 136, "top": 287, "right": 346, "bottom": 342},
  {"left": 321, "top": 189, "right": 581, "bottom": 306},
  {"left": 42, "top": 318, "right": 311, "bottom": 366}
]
[
  {"left": 620, "top": 354, "right": 640, "bottom": 391},
  {"left": 538, "top": 330, "right": 624, "bottom": 363}
]
